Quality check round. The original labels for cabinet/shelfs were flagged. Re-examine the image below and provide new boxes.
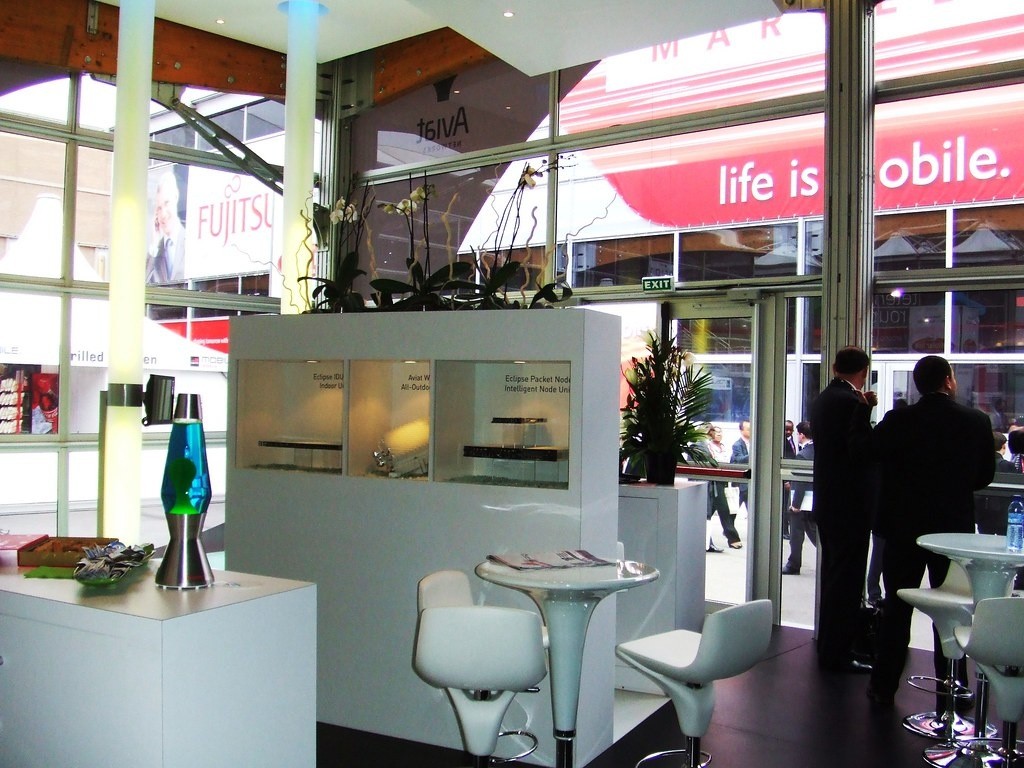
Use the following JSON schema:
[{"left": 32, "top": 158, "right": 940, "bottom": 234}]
[
  {"left": 616, "top": 480, "right": 708, "bottom": 697},
  {"left": 226, "top": 309, "right": 623, "bottom": 768}
]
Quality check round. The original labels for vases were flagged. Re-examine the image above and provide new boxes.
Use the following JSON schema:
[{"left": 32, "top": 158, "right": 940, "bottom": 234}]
[{"left": 644, "top": 443, "right": 678, "bottom": 486}]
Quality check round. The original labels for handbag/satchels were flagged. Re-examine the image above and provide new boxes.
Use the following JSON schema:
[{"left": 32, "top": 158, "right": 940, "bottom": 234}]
[{"left": 724, "top": 481, "right": 739, "bottom": 514}]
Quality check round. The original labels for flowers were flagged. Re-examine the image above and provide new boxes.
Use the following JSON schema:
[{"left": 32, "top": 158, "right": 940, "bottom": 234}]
[{"left": 619, "top": 333, "right": 720, "bottom": 485}]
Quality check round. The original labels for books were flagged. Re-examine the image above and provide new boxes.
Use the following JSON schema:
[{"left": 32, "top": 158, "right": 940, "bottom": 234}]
[{"left": 485, "top": 549, "right": 618, "bottom": 573}]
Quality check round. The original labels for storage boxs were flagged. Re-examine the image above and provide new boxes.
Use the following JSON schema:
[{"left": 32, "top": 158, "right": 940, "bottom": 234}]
[{"left": 0, "top": 534, "right": 119, "bottom": 567}]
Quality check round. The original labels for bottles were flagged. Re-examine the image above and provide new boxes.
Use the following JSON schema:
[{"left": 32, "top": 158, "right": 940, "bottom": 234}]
[{"left": 1006, "top": 495, "right": 1024, "bottom": 554}]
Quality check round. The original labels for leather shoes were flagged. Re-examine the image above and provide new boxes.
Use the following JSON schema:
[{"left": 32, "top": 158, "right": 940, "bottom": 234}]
[
  {"left": 866, "top": 683, "right": 894, "bottom": 710},
  {"left": 936, "top": 691, "right": 975, "bottom": 715},
  {"left": 819, "top": 658, "right": 873, "bottom": 674},
  {"left": 783, "top": 534, "right": 791, "bottom": 539},
  {"left": 782, "top": 567, "right": 800, "bottom": 575}
]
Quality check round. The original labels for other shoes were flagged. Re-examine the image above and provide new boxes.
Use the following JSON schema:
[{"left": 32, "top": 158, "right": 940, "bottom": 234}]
[
  {"left": 730, "top": 542, "right": 742, "bottom": 549},
  {"left": 868, "top": 596, "right": 884, "bottom": 606},
  {"left": 706, "top": 545, "right": 724, "bottom": 552}
]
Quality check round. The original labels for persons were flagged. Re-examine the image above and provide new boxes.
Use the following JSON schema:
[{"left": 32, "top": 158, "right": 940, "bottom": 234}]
[
  {"left": 145, "top": 171, "right": 188, "bottom": 284},
  {"left": 865, "top": 419, "right": 1024, "bottom": 608},
  {"left": 867, "top": 355, "right": 995, "bottom": 712},
  {"left": 810, "top": 345, "right": 878, "bottom": 669},
  {"left": 686, "top": 419, "right": 819, "bottom": 576}
]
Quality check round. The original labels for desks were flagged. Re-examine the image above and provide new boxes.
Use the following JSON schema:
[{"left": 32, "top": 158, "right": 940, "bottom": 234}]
[
  {"left": 917, "top": 533, "right": 1024, "bottom": 768},
  {"left": 475, "top": 558, "right": 661, "bottom": 768},
  {"left": 0, "top": 551, "right": 317, "bottom": 768}
]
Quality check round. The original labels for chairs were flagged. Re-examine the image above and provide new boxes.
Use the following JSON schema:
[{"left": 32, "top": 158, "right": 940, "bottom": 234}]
[
  {"left": 897, "top": 559, "right": 998, "bottom": 741},
  {"left": 953, "top": 597, "right": 1024, "bottom": 768},
  {"left": 417, "top": 570, "right": 550, "bottom": 649},
  {"left": 414, "top": 606, "right": 547, "bottom": 768},
  {"left": 616, "top": 599, "right": 773, "bottom": 768}
]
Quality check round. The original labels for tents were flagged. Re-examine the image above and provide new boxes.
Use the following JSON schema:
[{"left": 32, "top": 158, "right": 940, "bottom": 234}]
[{"left": 0, "top": 192, "right": 235, "bottom": 514}]
